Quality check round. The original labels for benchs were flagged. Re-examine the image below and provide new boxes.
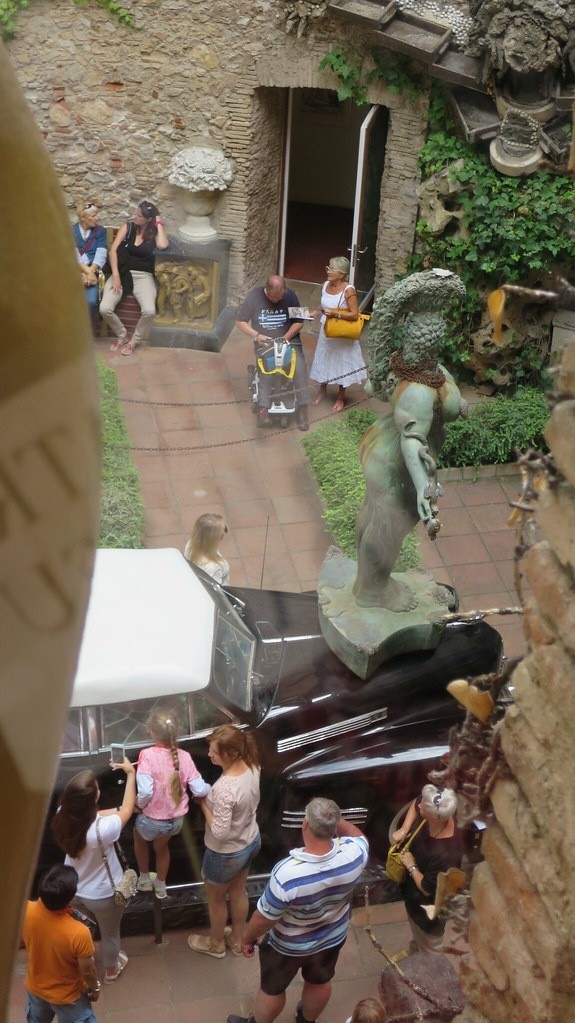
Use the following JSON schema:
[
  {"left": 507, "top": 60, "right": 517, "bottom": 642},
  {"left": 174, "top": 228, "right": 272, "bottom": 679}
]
[{"left": 103, "top": 225, "right": 142, "bottom": 339}]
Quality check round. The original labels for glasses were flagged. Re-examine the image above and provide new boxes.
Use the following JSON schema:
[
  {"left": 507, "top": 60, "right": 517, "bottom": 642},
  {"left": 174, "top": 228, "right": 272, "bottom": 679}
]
[
  {"left": 82, "top": 202, "right": 95, "bottom": 212},
  {"left": 325, "top": 266, "right": 340, "bottom": 272},
  {"left": 143, "top": 201, "right": 154, "bottom": 216},
  {"left": 222, "top": 525, "right": 228, "bottom": 534},
  {"left": 434, "top": 786, "right": 445, "bottom": 815}
]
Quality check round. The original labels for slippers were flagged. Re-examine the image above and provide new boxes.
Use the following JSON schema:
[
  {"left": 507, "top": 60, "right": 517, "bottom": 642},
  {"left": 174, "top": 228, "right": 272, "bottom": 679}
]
[
  {"left": 311, "top": 390, "right": 327, "bottom": 406},
  {"left": 120, "top": 342, "right": 136, "bottom": 355},
  {"left": 333, "top": 396, "right": 346, "bottom": 411},
  {"left": 110, "top": 338, "right": 129, "bottom": 352}
]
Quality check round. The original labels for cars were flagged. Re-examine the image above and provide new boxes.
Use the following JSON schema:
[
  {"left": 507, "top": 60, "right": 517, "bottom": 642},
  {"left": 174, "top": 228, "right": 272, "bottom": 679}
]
[{"left": 14, "top": 544, "right": 507, "bottom": 944}]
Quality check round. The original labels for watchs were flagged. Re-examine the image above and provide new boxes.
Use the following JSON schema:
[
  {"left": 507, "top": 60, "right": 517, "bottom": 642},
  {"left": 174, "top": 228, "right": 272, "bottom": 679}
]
[{"left": 408, "top": 865, "right": 418, "bottom": 874}]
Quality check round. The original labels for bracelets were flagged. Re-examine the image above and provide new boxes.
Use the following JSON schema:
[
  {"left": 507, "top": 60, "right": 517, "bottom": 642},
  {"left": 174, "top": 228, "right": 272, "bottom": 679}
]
[
  {"left": 398, "top": 829, "right": 406, "bottom": 836},
  {"left": 254, "top": 334, "right": 259, "bottom": 341},
  {"left": 135, "top": 804, "right": 142, "bottom": 810},
  {"left": 117, "top": 806, "right": 120, "bottom": 811},
  {"left": 95, "top": 981, "right": 100, "bottom": 991},
  {"left": 155, "top": 221, "right": 163, "bottom": 226}
]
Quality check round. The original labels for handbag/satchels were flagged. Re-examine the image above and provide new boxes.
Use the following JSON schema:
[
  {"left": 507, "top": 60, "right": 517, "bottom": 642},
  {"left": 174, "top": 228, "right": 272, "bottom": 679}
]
[
  {"left": 385, "top": 831, "right": 412, "bottom": 883},
  {"left": 107, "top": 222, "right": 136, "bottom": 266},
  {"left": 323, "top": 308, "right": 371, "bottom": 340},
  {"left": 115, "top": 869, "right": 138, "bottom": 907}
]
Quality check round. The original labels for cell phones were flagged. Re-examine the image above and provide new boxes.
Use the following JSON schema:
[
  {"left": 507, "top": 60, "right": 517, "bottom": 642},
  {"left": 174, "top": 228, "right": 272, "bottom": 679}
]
[{"left": 111, "top": 743, "right": 124, "bottom": 764}]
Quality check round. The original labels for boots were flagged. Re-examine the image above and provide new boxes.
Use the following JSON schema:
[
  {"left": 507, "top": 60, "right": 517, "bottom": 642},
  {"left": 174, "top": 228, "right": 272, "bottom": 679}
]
[
  {"left": 296, "top": 404, "right": 309, "bottom": 431},
  {"left": 256, "top": 408, "right": 272, "bottom": 428}
]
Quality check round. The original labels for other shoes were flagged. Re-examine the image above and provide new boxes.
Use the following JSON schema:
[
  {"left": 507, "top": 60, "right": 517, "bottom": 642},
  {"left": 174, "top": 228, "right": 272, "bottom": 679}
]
[{"left": 387, "top": 949, "right": 408, "bottom": 965}]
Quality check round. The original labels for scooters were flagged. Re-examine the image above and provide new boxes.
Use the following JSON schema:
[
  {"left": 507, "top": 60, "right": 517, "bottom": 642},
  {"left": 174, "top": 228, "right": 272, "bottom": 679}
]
[{"left": 246, "top": 334, "right": 304, "bottom": 430}]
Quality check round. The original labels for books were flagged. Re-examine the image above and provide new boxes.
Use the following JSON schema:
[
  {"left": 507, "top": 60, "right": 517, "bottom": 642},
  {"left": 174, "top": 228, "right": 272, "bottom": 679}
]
[{"left": 288, "top": 307, "right": 314, "bottom": 320}]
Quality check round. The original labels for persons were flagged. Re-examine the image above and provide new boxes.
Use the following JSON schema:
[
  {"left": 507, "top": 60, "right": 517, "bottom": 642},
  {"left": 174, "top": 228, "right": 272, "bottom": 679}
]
[
  {"left": 391, "top": 784, "right": 462, "bottom": 961},
  {"left": 188, "top": 726, "right": 262, "bottom": 958},
  {"left": 353, "top": 314, "right": 460, "bottom": 613},
  {"left": 237, "top": 275, "right": 311, "bottom": 431},
  {"left": 52, "top": 757, "right": 136, "bottom": 985},
  {"left": 184, "top": 514, "right": 229, "bottom": 586},
  {"left": 71, "top": 203, "right": 108, "bottom": 313},
  {"left": 99, "top": 201, "right": 169, "bottom": 356},
  {"left": 308, "top": 257, "right": 367, "bottom": 410},
  {"left": 133, "top": 708, "right": 211, "bottom": 899},
  {"left": 227, "top": 798, "right": 369, "bottom": 1023},
  {"left": 21, "top": 865, "right": 100, "bottom": 1023},
  {"left": 347, "top": 998, "right": 385, "bottom": 1023}
]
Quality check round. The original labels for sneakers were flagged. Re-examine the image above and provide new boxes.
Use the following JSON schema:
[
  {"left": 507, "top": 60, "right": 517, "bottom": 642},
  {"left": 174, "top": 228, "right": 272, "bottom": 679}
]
[
  {"left": 228, "top": 1014, "right": 257, "bottom": 1023},
  {"left": 149, "top": 871, "right": 166, "bottom": 898},
  {"left": 295, "top": 1000, "right": 315, "bottom": 1023},
  {"left": 136, "top": 877, "right": 153, "bottom": 891}
]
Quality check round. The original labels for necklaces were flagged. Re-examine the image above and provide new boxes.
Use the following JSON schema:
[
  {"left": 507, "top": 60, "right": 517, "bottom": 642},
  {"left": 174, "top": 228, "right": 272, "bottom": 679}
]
[{"left": 432, "top": 821, "right": 448, "bottom": 838}]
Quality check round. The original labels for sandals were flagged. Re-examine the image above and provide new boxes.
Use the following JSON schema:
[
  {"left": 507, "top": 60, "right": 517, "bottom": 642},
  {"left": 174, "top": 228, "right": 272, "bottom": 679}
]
[
  {"left": 187, "top": 934, "right": 226, "bottom": 958},
  {"left": 103, "top": 949, "right": 128, "bottom": 984},
  {"left": 224, "top": 927, "right": 244, "bottom": 956}
]
